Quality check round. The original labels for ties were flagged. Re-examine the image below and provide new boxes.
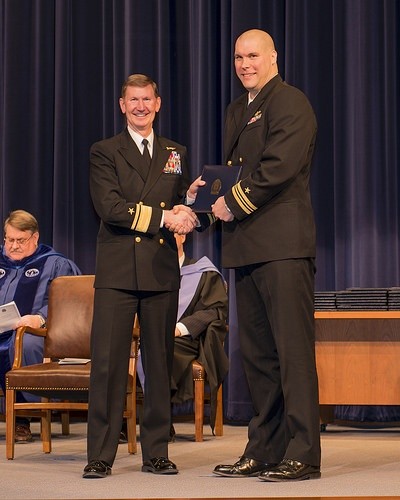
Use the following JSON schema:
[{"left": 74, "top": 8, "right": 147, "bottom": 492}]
[{"left": 142, "top": 138, "right": 152, "bottom": 168}]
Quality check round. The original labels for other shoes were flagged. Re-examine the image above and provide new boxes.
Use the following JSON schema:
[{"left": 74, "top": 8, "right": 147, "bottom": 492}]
[
  {"left": 167, "top": 427, "right": 176, "bottom": 443},
  {"left": 118, "top": 431, "right": 128, "bottom": 444}
]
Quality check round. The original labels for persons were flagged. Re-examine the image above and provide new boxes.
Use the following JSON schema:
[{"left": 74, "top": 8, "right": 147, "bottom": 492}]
[
  {"left": 0, "top": 208, "right": 82, "bottom": 444},
  {"left": 166, "top": 29, "right": 322, "bottom": 481},
  {"left": 83, "top": 74, "right": 207, "bottom": 478},
  {"left": 118, "top": 232, "right": 230, "bottom": 443}
]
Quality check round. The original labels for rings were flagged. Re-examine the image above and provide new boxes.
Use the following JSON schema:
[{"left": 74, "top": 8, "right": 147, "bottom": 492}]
[{"left": 180, "top": 227, "right": 185, "bottom": 229}]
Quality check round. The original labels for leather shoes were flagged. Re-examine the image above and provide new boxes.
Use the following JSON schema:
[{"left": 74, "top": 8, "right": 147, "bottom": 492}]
[
  {"left": 213, "top": 456, "right": 276, "bottom": 478},
  {"left": 82, "top": 460, "right": 111, "bottom": 478},
  {"left": 256, "top": 458, "right": 321, "bottom": 482},
  {"left": 15, "top": 424, "right": 32, "bottom": 444},
  {"left": 142, "top": 456, "right": 178, "bottom": 475}
]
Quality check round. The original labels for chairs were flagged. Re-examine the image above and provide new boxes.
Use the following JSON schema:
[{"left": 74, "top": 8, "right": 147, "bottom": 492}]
[
  {"left": 5, "top": 275, "right": 136, "bottom": 460},
  {"left": 136, "top": 280, "right": 229, "bottom": 442}
]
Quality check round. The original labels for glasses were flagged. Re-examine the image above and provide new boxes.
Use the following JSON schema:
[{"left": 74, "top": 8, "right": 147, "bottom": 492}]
[{"left": 3, "top": 233, "right": 34, "bottom": 245}]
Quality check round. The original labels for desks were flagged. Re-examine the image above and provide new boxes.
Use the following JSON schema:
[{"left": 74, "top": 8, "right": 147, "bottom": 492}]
[{"left": 314, "top": 311, "right": 400, "bottom": 432}]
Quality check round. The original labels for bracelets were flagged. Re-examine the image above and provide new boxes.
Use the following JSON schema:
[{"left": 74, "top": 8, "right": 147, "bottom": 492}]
[{"left": 225, "top": 204, "right": 230, "bottom": 212}]
